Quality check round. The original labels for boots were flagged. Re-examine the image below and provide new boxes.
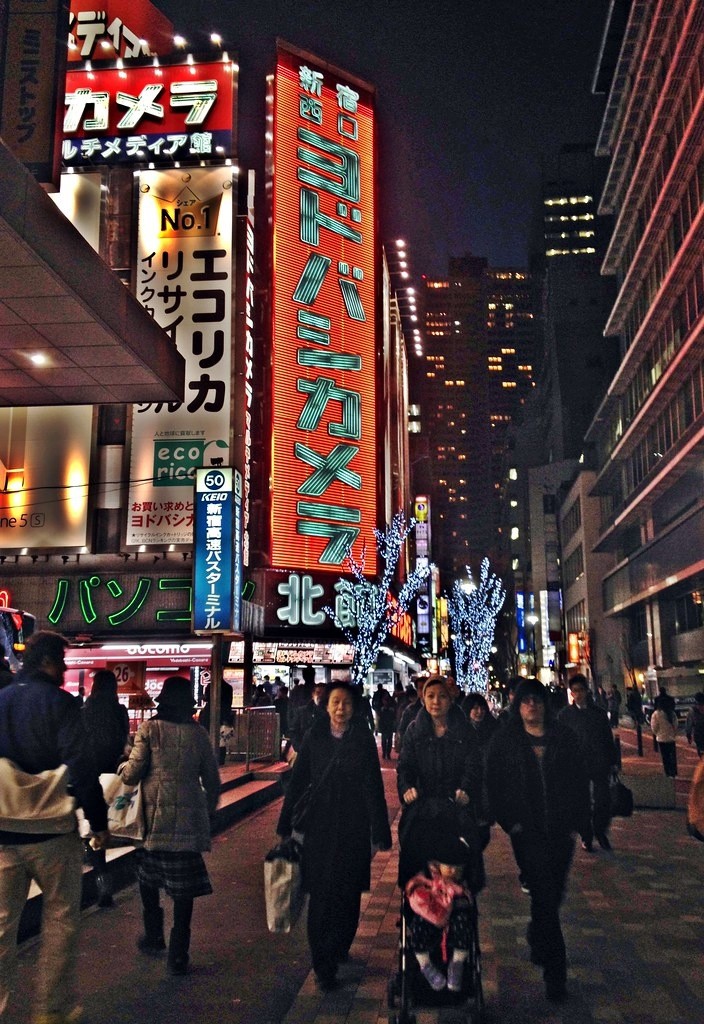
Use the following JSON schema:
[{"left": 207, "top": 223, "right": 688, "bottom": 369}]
[
  {"left": 136, "top": 908, "right": 166, "bottom": 953},
  {"left": 167, "top": 925, "right": 192, "bottom": 976}
]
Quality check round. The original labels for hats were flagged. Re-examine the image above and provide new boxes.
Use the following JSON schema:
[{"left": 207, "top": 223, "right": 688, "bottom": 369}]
[{"left": 422, "top": 676, "right": 456, "bottom": 706}]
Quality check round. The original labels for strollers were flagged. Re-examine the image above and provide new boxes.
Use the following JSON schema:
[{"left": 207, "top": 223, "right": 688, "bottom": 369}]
[{"left": 384, "top": 790, "right": 486, "bottom": 1024}]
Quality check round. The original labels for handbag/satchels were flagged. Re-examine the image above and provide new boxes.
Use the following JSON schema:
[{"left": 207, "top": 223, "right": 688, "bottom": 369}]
[
  {"left": 263, "top": 838, "right": 306, "bottom": 933},
  {"left": 609, "top": 772, "right": 634, "bottom": 816}
]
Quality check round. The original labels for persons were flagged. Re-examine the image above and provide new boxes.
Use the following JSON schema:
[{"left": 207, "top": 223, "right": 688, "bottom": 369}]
[
  {"left": 481, "top": 678, "right": 592, "bottom": 982},
  {"left": 409, "top": 843, "right": 477, "bottom": 993},
  {"left": 556, "top": 675, "right": 619, "bottom": 853},
  {"left": 253, "top": 675, "right": 326, "bottom": 759},
  {"left": 0, "top": 628, "right": 222, "bottom": 1024},
  {"left": 597, "top": 682, "right": 704, "bottom": 779},
  {"left": 395, "top": 677, "right": 466, "bottom": 754},
  {"left": 398, "top": 673, "right": 486, "bottom": 894},
  {"left": 276, "top": 677, "right": 393, "bottom": 992},
  {"left": 356, "top": 682, "right": 417, "bottom": 760},
  {"left": 460, "top": 675, "right": 524, "bottom": 853}
]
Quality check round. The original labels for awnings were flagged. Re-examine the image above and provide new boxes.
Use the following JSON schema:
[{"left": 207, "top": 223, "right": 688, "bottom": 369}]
[{"left": 62, "top": 643, "right": 217, "bottom": 669}]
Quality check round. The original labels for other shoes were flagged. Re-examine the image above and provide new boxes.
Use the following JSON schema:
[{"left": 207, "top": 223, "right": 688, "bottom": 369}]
[
  {"left": 420, "top": 964, "right": 447, "bottom": 991},
  {"left": 596, "top": 831, "right": 610, "bottom": 851},
  {"left": 582, "top": 838, "right": 591, "bottom": 851},
  {"left": 543, "top": 966, "right": 567, "bottom": 999},
  {"left": 448, "top": 960, "right": 465, "bottom": 992},
  {"left": 38, "top": 1004, "right": 83, "bottom": 1024},
  {"left": 522, "top": 885, "right": 531, "bottom": 895}
]
